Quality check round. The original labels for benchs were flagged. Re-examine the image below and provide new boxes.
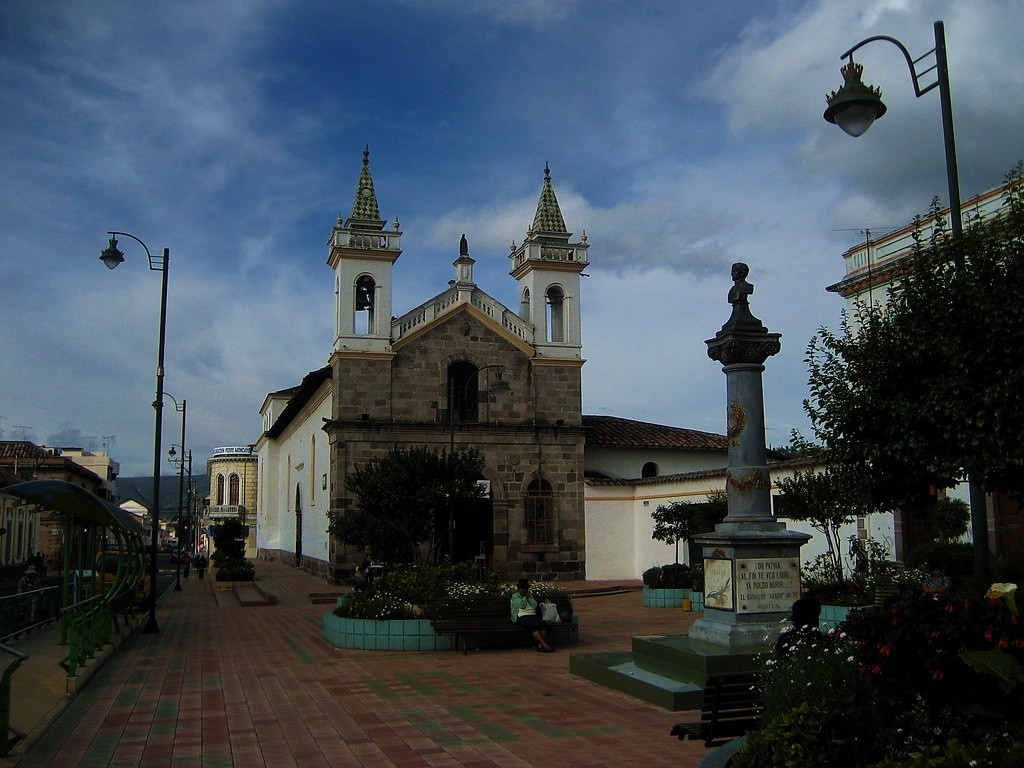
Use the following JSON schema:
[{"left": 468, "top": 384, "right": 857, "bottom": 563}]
[
  {"left": 670, "top": 674, "right": 765, "bottom": 749},
  {"left": 430, "top": 598, "right": 578, "bottom": 654}
]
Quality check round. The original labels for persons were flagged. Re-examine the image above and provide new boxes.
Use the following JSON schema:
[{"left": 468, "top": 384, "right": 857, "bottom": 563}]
[
  {"left": 510, "top": 578, "right": 556, "bottom": 653},
  {"left": 27, "top": 551, "right": 48, "bottom": 577},
  {"left": 18, "top": 565, "right": 45, "bottom": 634},
  {"left": 183, "top": 552, "right": 207, "bottom": 580}
]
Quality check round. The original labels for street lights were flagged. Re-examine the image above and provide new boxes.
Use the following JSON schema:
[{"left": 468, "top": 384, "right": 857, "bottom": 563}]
[
  {"left": 98, "top": 231, "right": 169, "bottom": 634},
  {"left": 150, "top": 390, "right": 187, "bottom": 591},
  {"left": 823, "top": 17, "right": 992, "bottom": 597},
  {"left": 169, "top": 443, "right": 193, "bottom": 579}
]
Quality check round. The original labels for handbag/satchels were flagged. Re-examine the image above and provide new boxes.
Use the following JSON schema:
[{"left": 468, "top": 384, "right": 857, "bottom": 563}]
[{"left": 539, "top": 599, "right": 560, "bottom": 623}]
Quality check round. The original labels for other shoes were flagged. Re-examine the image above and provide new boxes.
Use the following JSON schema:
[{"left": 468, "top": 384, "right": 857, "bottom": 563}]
[{"left": 536, "top": 646, "right": 555, "bottom": 652}]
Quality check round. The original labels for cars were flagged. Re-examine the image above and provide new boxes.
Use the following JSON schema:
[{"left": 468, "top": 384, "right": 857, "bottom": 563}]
[{"left": 94, "top": 550, "right": 168, "bottom": 606}]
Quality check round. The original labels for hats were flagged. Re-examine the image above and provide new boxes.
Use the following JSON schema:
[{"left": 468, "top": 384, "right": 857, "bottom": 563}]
[
  {"left": 515, "top": 579, "right": 531, "bottom": 588},
  {"left": 24, "top": 566, "right": 36, "bottom": 573}
]
